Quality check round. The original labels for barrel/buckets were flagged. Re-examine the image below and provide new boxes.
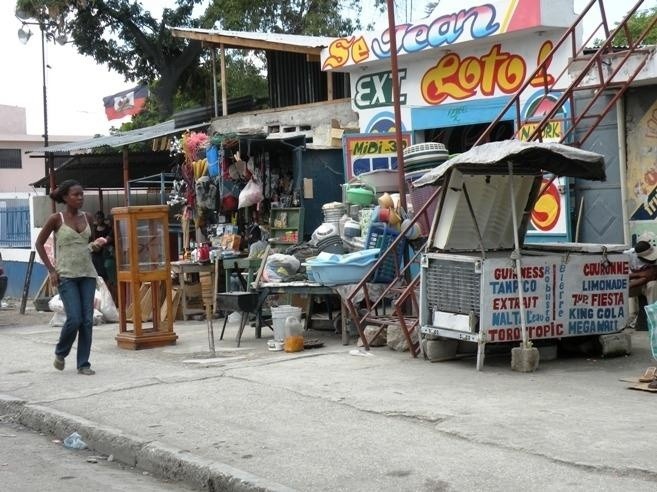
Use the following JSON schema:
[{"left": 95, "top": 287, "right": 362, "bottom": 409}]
[
  {"left": 284, "top": 317, "right": 304, "bottom": 352},
  {"left": 271, "top": 306, "right": 301, "bottom": 341},
  {"left": 316, "top": 235, "right": 345, "bottom": 255}
]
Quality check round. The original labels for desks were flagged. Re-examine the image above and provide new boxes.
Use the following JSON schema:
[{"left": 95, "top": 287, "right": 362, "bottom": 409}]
[{"left": 160, "top": 258, "right": 390, "bottom": 346}]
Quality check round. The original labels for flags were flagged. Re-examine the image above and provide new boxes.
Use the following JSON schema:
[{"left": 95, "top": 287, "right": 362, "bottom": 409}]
[{"left": 102, "top": 82, "right": 149, "bottom": 120}]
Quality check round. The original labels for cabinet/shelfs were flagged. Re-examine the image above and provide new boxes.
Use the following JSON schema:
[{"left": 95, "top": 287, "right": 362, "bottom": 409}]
[{"left": 266, "top": 206, "right": 305, "bottom": 248}]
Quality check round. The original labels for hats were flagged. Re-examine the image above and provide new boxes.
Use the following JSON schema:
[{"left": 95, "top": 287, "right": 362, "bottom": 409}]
[{"left": 633, "top": 241, "right": 657, "bottom": 262}]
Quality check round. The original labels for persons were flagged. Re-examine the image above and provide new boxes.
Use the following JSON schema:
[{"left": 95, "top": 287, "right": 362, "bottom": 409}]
[
  {"left": 35, "top": 179, "right": 103, "bottom": 377},
  {"left": 88, "top": 209, "right": 113, "bottom": 269},
  {"left": 229, "top": 226, "right": 273, "bottom": 293},
  {"left": 622, "top": 239, "right": 656, "bottom": 329}
]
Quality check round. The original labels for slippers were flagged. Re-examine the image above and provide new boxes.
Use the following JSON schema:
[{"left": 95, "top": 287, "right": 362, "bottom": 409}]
[
  {"left": 53, "top": 355, "right": 64, "bottom": 371},
  {"left": 77, "top": 366, "right": 96, "bottom": 375},
  {"left": 639, "top": 367, "right": 656, "bottom": 389}
]
